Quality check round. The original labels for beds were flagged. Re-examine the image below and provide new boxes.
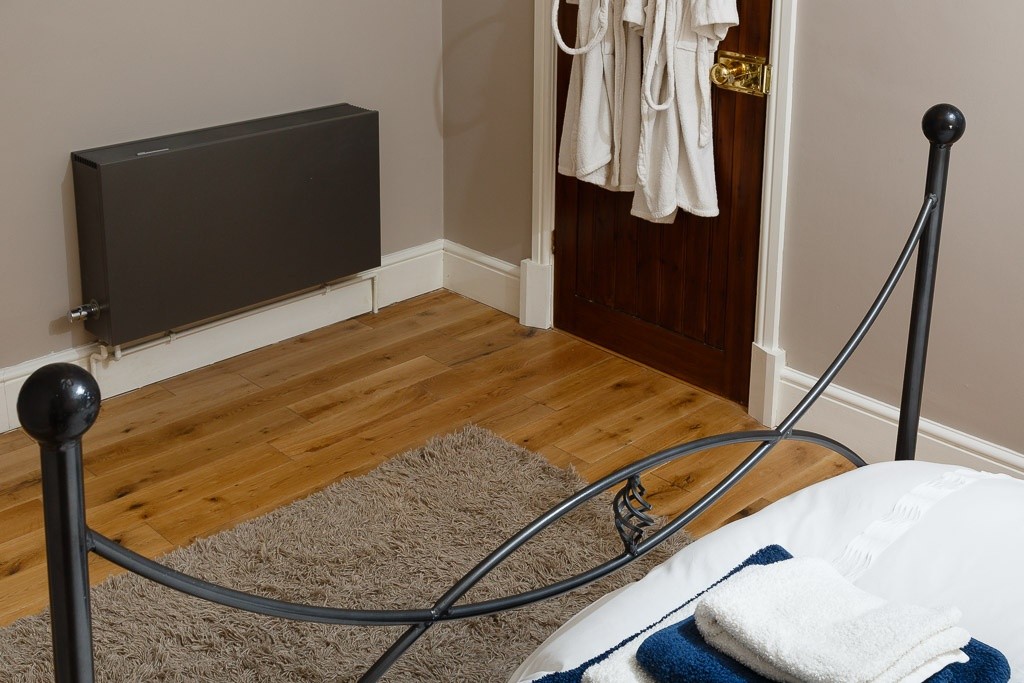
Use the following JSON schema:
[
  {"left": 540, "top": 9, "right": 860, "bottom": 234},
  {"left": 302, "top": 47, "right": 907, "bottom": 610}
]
[{"left": 18, "top": 104, "right": 1024, "bottom": 682}]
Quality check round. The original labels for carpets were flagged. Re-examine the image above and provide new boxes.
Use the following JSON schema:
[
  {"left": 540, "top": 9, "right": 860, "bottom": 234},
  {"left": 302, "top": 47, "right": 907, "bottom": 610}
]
[{"left": 1, "top": 424, "right": 696, "bottom": 683}]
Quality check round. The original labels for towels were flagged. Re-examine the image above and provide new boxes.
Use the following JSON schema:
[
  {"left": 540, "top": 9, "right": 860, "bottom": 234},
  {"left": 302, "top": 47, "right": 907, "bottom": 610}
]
[
  {"left": 636, "top": 543, "right": 1009, "bottom": 682},
  {"left": 694, "top": 557, "right": 972, "bottom": 682}
]
